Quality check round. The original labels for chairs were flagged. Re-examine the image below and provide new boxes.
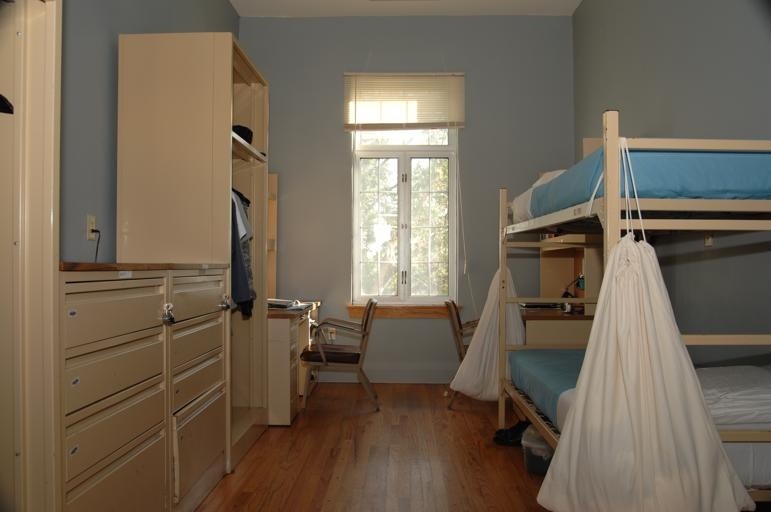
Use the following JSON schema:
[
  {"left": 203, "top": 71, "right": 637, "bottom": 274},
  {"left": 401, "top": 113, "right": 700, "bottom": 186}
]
[
  {"left": 300, "top": 298, "right": 381, "bottom": 414},
  {"left": 445, "top": 299, "right": 482, "bottom": 409}
]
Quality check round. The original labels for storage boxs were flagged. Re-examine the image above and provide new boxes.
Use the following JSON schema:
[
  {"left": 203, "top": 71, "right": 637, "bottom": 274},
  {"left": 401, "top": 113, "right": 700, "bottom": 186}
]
[{"left": 522, "top": 424, "right": 555, "bottom": 475}]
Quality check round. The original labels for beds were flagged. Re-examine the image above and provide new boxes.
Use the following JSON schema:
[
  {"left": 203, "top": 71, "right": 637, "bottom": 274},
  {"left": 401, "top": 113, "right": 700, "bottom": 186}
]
[{"left": 496, "top": 110, "right": 771, "bottom": 512}]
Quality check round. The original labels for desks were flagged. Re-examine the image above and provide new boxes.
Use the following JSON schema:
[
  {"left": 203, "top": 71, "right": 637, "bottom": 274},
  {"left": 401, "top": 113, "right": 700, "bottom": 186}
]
[
  {"left": 268, "top": 300, "right": 322, "bottom": 426},
  {"left": 521, "top": 233, "right": 603, "bottom": 344}
]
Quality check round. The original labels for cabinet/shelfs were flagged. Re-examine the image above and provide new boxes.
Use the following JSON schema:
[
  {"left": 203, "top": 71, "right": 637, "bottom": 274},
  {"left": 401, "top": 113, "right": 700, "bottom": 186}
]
[{"left": 115, "top": 32, "right": 271, "bottom": 474}]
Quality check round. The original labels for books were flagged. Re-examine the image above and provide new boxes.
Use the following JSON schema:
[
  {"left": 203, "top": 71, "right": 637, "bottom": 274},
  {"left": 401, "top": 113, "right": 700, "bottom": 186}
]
[
  {"left": 518, "top": 302, "right": 559, "bottom": 308},
  {"left": 268, "top": 297, "right": 294, "bottom": 308}
]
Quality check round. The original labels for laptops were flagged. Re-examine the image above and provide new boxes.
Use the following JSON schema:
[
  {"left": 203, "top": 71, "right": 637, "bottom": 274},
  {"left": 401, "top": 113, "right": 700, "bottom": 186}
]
[{"left": 518, "top": 301, "right": 561, "bottom": 309}]
